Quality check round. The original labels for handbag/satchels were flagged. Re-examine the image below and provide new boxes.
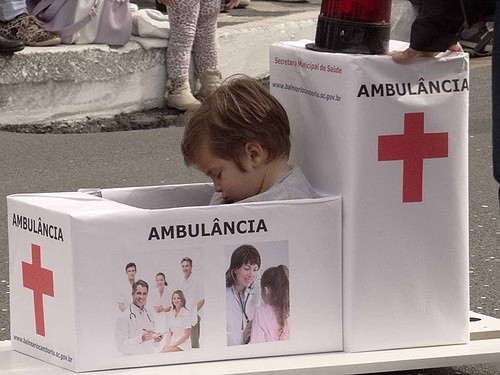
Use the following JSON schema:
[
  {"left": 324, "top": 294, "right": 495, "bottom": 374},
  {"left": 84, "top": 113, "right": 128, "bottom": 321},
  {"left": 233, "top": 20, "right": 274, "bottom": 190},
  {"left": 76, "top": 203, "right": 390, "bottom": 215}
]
[{"left": 31, "top": 0, "right": 132, "bottom": 46}]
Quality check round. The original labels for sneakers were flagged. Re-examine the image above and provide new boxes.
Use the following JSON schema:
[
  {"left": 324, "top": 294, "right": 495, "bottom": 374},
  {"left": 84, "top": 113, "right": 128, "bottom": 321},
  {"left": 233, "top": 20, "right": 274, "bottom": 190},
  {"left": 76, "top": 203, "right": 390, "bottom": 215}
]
[{"left": 0, "top": 13, "right": 61, "bottom": 46}]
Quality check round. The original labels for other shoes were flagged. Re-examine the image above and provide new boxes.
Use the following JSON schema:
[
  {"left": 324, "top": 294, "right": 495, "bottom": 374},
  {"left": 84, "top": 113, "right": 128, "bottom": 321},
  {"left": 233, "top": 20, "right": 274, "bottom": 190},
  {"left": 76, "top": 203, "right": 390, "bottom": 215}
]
[
  {"left": 166, "top": 85, "right": 201, "bottom": 110},
  {"left": 194, "top": 69, "right": 221, "bottom": 96},
  {"left": 459, "top": 25, "right": 493, "bottom": 56},
  {"left": 226, "top": 0, "right": 250, "bottom": 8},
  {"left": 0, "top": 35, "right": 24, "bottom": 53}
]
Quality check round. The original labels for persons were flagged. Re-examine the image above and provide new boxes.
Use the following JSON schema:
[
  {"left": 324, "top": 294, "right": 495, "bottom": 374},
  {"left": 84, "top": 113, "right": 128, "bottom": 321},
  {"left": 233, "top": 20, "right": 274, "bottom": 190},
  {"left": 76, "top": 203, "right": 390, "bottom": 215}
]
[
  {"left": 0, "top": 0, "right": 62, "bottom": 47},
  {"left": 391, "top": 0, "right": 499, "bottom": 194},
  {"left": 156, "top": 0, "right": 251, "bottom": 112},
  {"left": 179, "top": 72, "right": 323, "bottom": 207},
  {"left": 117, "top": 256, "right": 205, "bottom": 355},
  {"left": 0, "top": 35, "right": 25, "bottom": 56},
  {"left": 226, "top": 244, "right": 265, "bottom": 347},
  {"left": 248, "top": 263, "right": 294, "bottom": 343}
]
